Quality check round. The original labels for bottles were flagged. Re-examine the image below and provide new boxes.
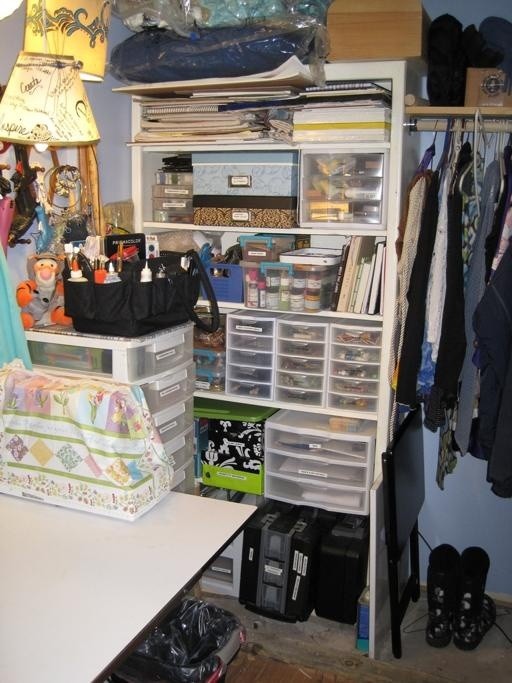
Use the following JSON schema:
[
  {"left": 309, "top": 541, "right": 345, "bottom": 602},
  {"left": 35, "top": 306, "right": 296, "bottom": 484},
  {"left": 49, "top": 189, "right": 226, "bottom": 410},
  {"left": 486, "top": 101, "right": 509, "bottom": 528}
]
[
  {"left": 139, "top": 260, "right": 153, "bottom": 283},
  {"left": 354, "top": 585, "right": 370, "bottom": 653},
  {"left": 66, "top": 259, "right": 89, "bottom": 282},
  {"left": 94, "top": 262, "right": 122, "bottom": 285}
]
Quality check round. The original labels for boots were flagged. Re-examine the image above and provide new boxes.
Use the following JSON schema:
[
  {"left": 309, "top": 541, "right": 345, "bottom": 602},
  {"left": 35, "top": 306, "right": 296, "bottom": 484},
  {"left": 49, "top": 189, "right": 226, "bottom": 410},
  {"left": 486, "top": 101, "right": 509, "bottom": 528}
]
[
  {"left": 423, "top": 542, "right": 460, "bottom": 649},
  {"left": 456, "top": 547, "right": 496, "bottom": 651}
]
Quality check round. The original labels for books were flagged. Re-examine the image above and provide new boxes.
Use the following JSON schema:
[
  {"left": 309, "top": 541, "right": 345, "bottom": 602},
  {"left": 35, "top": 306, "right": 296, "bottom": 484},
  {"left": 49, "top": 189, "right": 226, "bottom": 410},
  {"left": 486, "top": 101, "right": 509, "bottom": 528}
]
[
  {"left": 328, "top": 235, "right": 386, "bottom": 316},
  {"left": 112, "top": 54, "right": 391, "bottom": 145}
]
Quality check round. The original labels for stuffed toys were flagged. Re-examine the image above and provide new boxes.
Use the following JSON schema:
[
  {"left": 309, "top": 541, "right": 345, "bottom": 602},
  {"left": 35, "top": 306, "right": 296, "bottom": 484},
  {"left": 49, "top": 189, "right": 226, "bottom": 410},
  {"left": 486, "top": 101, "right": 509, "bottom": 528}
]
[{"left": 16, "top": 253, "right": 73, "bottom": 329}]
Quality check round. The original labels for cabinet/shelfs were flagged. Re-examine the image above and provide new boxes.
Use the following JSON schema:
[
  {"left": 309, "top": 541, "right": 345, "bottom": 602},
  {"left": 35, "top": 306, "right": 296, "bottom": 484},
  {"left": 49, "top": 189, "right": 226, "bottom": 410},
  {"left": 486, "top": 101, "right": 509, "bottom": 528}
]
[{"left": 112, "top": 60, "right": 419, "bottom": 660}]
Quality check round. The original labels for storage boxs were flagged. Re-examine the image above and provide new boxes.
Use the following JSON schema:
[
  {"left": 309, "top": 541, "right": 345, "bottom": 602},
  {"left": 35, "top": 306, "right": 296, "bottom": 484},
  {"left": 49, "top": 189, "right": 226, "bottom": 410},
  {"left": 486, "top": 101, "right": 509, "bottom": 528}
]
[
  {"left": 236, "top": 231, "right": 296, "bottom": 262},
  {"left": 316, "top": 512, "right": 370, "bottom": 626},
  {"left": 264, "top": 409, "right": 377, "bottom": 516},
  {"left": 298, "top": 146, "right": 386, "bottom": 230},
  {"left": 26, "top": 320, "right": 196, "bottom": 495},
  {"left": 195, "top": 311, "right": 226, "bottom": 394},
  {"left": 225, "top": 310, "right": 285, "bottom": 402},
  {"left": 325, "top": 0, "right": 432, "bottom": 78},
  {"left": 192, "top": 151, "right": 296, "bottom": 227},
  {"left": 239, "top": 257, "right": 339, "bottom": 314},
  {"left": 275, "top": 312, "right": 337, "bottom": 405},
  {"left": 196, "top": 401, "right": 275, "bottom": 495},
  {"left": 239, "top": 497, "right": 320, "bottom": 622},
  {"left": 326, "top": 319, "right": 381, "bottom": 417}
]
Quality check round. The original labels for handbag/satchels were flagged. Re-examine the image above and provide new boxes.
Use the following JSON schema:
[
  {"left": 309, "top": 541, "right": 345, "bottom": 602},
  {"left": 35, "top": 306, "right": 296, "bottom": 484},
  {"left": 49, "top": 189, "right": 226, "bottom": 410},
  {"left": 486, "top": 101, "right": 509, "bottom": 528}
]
[{"left": 61, "top": 248, "right": 220, "bottom": 335}]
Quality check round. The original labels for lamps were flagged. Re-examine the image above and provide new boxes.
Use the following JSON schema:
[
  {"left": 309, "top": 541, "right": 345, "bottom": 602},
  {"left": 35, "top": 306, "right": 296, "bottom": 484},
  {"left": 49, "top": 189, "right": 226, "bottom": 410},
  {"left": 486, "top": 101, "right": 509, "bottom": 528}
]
[{"left": 0, "top": 51, "right": 103, "bottom": 153}]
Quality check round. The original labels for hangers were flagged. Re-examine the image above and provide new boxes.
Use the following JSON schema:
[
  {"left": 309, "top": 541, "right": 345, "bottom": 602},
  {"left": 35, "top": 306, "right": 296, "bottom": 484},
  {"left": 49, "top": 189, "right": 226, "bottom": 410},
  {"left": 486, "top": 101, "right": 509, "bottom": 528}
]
[{"left": 428, "top": 110, "right": 511, "bottom": 219}]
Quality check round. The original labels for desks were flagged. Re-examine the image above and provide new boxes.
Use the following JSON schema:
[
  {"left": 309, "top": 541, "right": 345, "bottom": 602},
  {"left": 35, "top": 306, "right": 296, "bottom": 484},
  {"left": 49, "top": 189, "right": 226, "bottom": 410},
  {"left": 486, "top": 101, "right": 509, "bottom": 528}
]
[{"left": 0, "top": 485, "right": 261, "bottom": 683}]
